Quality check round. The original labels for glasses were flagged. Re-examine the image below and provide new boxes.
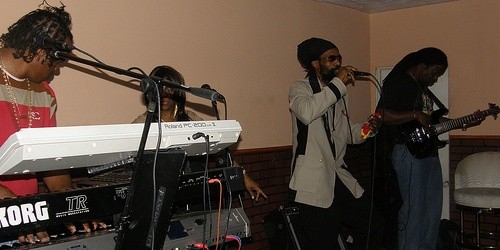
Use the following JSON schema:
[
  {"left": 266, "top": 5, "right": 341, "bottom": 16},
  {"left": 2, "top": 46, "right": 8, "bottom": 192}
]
[{"left": 318, "top": 54, "right": 342, "bottom": 62}]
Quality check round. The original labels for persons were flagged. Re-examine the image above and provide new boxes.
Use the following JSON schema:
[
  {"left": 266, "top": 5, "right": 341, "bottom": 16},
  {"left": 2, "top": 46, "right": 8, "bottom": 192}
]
[
  {"left": 375, "top": 47, "right": 486, "bottom": 250},
  {"left": 132, "top": 65, "right": 268, "bottom": 200},
  {"left": 288, "top": 37, "right": 381, "bottom": 250},
  {"left": 0, "top": 0, "right": 107, "bottom": 244}
]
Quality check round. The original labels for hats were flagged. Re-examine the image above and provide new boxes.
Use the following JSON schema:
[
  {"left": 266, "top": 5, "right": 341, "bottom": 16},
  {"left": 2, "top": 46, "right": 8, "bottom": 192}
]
[{"left": 297, "top": 38, "right": 337, "bottom": 70}]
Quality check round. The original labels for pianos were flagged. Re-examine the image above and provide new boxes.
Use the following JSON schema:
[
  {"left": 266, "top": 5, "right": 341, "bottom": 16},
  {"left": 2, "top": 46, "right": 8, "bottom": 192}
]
[{"left": 0, "top": 119, "right": 251, "bottom": 250}]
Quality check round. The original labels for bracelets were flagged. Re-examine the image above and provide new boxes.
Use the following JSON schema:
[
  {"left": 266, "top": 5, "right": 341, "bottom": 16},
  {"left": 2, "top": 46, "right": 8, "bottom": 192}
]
[{"left": 243, "top": 169, "right": 246, "bottom": 174}]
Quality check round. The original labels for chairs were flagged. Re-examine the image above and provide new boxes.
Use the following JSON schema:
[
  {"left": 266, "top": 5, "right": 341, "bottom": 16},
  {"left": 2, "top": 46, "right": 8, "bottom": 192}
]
[{"left": 454, "top": 151, "right": 500, "bottom": 250}]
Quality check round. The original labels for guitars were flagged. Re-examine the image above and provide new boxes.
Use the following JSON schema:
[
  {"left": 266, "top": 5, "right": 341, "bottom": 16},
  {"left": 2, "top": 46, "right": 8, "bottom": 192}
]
[{"left": 406, "top": 103, "right": 500, "bottom": 159}]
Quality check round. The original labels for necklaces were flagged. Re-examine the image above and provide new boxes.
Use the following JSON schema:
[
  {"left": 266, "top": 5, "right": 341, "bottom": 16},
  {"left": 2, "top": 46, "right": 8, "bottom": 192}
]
[{"left": 0, "top": 57, "right": 32, "bottom": 128}]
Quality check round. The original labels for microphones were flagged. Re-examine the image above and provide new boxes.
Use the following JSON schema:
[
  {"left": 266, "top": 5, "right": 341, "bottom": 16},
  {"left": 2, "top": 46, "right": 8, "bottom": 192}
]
[
  {"left": 200, "top": 83, "right": 225, "bottom": 103},
  {"left": 49, "top": 51, "right": 61, "bottom": 58},
  {"left": 43, "top": 36, "right": 72, "bottom": 50},
  {"left": 335, "top": 69, "right": 372, "bottom": 77}
]
